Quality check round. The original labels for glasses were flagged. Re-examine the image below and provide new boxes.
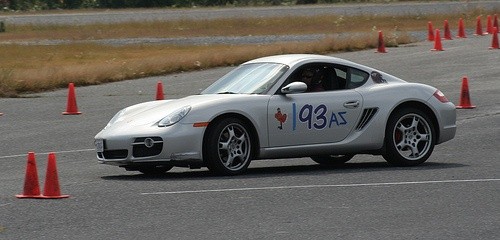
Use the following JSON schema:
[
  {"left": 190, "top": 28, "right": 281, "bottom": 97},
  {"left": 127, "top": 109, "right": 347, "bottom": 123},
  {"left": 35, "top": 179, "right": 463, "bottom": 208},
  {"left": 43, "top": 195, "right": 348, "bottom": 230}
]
[{"left": 301, "top": 75, "right": 312, "bottom": 78}]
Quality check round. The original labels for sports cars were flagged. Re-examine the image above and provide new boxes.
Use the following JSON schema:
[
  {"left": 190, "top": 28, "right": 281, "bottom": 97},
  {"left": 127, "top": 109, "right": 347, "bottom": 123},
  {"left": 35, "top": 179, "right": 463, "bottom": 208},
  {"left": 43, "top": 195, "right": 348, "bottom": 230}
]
[{"left": 94, "top": 54, "right": 457, "bottom": 175}]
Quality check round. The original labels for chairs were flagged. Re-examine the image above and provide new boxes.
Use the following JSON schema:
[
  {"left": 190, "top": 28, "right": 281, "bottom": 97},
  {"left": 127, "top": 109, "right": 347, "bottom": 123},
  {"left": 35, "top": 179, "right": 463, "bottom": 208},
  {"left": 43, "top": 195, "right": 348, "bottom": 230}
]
[{"left": 314, "top": 67, "right": 338, "bottom": 91}]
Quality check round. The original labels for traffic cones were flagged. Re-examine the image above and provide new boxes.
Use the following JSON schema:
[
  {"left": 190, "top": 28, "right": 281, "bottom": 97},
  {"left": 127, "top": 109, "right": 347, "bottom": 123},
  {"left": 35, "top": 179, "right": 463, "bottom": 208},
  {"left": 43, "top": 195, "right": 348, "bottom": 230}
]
[
  {"left": 442, "top": 20, "right": 453, "bottom": 40},
  {"left": 488, "top": 26, "right": 500, "bottom": 49},
  {"left": 473, "top": 14, "right": 500, "bottom": 35},
  {"left": 37, "top": 152, "right": 69, "bottom": 199},
  {"left": 15, "top": 151, "right": 42, "bottom": 198},
  {"left": 454, "top": 18, "right": 468, "bottom": 38},
  {"left": 430, "top": 29, "right": 445, "bottom": 51},
  {"left": 62, "top": 82, "right": 82, "bottom": 115},
  {"left": 455, "top": 77, "right": 476, "bottom": 108},
  {"left": 426, "top": 21, "right": 436, "bottom": 41},
  {"left": 374, "top": 31, "right": 388, "bottom": 53},
  {"left": 155, "top": 81, "right": 164, "bottom": 100}
]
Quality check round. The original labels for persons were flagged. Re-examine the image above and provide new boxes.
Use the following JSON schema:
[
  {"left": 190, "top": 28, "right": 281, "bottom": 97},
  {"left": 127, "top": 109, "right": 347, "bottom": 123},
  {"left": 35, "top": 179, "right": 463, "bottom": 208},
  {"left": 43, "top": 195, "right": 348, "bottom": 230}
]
[{"left": 282, "top": 67, "right": 324, "bottom": 92}]
